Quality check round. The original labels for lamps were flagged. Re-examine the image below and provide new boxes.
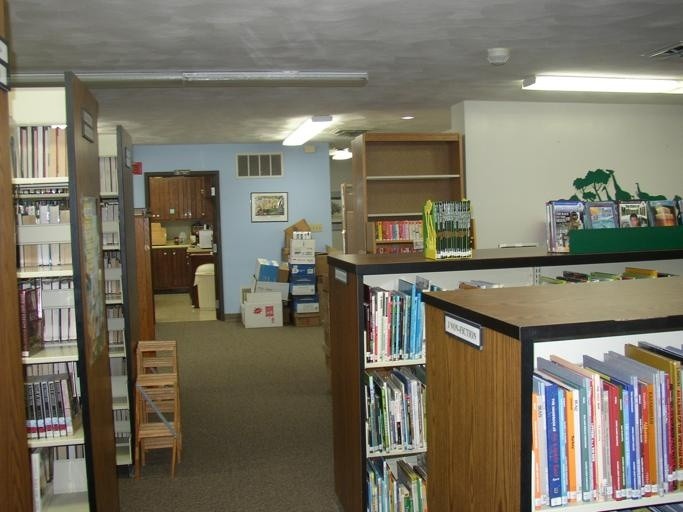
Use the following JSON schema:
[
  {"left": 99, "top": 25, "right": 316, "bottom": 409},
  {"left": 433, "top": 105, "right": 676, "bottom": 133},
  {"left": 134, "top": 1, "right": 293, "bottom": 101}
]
[
  {"left": 279, "top": 115, "right": 332, "bottom": 148},
  {"left": 518, "top": 71, "right": 682, "bottom": 97}
]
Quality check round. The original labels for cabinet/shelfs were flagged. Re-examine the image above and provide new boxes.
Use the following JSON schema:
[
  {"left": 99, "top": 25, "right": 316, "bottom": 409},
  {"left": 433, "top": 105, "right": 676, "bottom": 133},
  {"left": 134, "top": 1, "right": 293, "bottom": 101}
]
[
  {"left": 93, "top": 117, "right": 151, "bottom": 479},
  {"left": 201, "top": 176, "right": 215, "bottom": 221},
  {"left": 168, "top": 176, "right": 201, "bottom": 221},
  {"left": 131, "top": 206, "right": 162, "bottom": 388},
  {"left": 151, "top": 247, "right": 193, "bottom": 291},
  {"left": 350, "top": 132, "right": 464, "bottom": 255},
  {"left": 186, "top": 251, "right": 217, "bottom": 311},
  {"left": 147, "top": 176, "right": 168, "bottom": 222},
  {"left": 0, "top": 0, "right": 43, "bottom": 511},
  {"left": 322, "top": 243, "right": 683, "bottom": 512},
  {"left": 416, "top": 272, "right": 683, "bottom": 511},
  {"left": 8, "top": 67, "right": 129, "bottom": 511}
]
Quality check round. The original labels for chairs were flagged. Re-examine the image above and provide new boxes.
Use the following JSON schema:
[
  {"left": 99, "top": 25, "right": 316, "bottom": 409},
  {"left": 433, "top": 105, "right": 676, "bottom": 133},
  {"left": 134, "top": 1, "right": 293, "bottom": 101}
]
[{"left": 132, "top": 339, "right": 184, "bottom": 482}]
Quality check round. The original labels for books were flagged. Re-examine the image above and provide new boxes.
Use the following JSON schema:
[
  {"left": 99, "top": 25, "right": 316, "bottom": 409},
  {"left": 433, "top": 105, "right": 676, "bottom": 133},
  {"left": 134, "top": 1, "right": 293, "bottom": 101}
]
[
  {"left": 426, "top": 199, "right": 473, "bottom": 260},
  {"left": 25, "top": 363, "right": 84, "bottom": 441},
  {"left": 608, "top": 503, "right": 683, "bottom": 512},
  {"left": 30, "top": 447, "right": 54, "bottom": 512},
  {"left": 14, "top": 126, "right": 69, "bottom": 178},
  {"left": 99, "top": 199, "right": 119, "bottom": 245},
  {"left": 55, "top": 445, "right": 84, "bottom": 460},
  {"left": 364, "top": 366, "right": 429, "bottom": 454},
  {"left": 113, "top": 409, "right": 130, "bottom": 443},
  {"left": 533, "top": 339, "right": 683, "bottom": 510},
  {"left": 15, "top": 202, "right": 72, "bottom": 270},
  {"left": 99, "top": 157, "right": 117, "bottom": 194},
  {"left": 372, "top": 218, "right": 426, "bottom": 255},
  {"left": 17, "top": 277, "right": 78, "bottom": 358},
  {"left": 545, "top": 198, "right": 683, "bottom": 254},
  {"left": 541, "top": 264, "right": 673, "bottom": 285},
  {"left": 366, "top": 455, "right": 428, "bottom": 512},
  {"left": 459, "top": 278, "right": 504, "bottom": 289},
  {"left": 363, "top": 276, "right": 441, "bottom": 363},
  {"left": 102, "top": 251, "right": 122, "bottom": 294},
  {"left": 104, "top": 305, "right": 125, "bottom": 353}
]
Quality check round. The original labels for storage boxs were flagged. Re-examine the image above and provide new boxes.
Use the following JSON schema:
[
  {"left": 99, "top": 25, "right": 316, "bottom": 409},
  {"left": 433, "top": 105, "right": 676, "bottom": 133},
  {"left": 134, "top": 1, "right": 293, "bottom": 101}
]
[{"left": 238, "top": 219, "right": 331, "bottom": 330}]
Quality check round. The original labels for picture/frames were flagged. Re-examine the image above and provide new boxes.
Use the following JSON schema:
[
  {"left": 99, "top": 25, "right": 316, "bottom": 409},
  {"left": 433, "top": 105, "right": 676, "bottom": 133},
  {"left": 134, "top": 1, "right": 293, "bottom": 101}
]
[
  {"left": 249, "top": 191, "right": 289, "bottom": 224},
  {"left": 330, "top": 197, "right": 343, "bottom": 225}
]
